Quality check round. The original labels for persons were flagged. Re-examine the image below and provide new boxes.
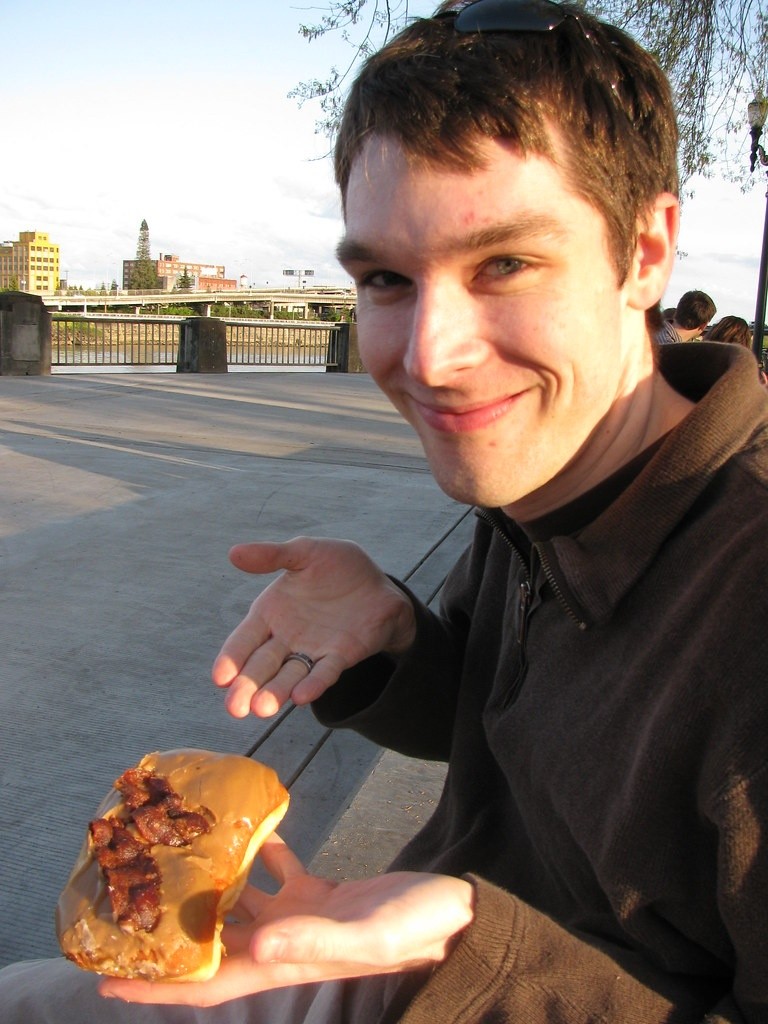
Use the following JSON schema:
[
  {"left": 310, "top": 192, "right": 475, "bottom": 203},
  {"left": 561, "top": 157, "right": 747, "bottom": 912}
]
[
  {"left": 654, "top": 291, "right": 716, "bottom": 345},
  {"left": 703, "top": 316, "right": 752, "bottom": 349},
  {"left": 0, "top": 0, "right": 768, "bottom": 1024}
]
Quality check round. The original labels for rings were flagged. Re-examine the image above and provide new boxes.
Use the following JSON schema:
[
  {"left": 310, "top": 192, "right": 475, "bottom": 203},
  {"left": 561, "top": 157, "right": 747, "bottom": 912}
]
[{"left": 282, "top": 652, "right": 314, "bottom": 672}]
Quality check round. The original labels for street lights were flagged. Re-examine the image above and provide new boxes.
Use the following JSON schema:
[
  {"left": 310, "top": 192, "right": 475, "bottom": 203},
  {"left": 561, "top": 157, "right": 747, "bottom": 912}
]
[{"left": 747, "top": 88, "right": 768, "bottom": 361}]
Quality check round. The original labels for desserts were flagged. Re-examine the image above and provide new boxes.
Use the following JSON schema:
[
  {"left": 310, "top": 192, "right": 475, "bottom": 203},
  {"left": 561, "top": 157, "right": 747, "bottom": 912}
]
[{"left": 54, "top": 747, "right": 291, "bottom": 983}]
